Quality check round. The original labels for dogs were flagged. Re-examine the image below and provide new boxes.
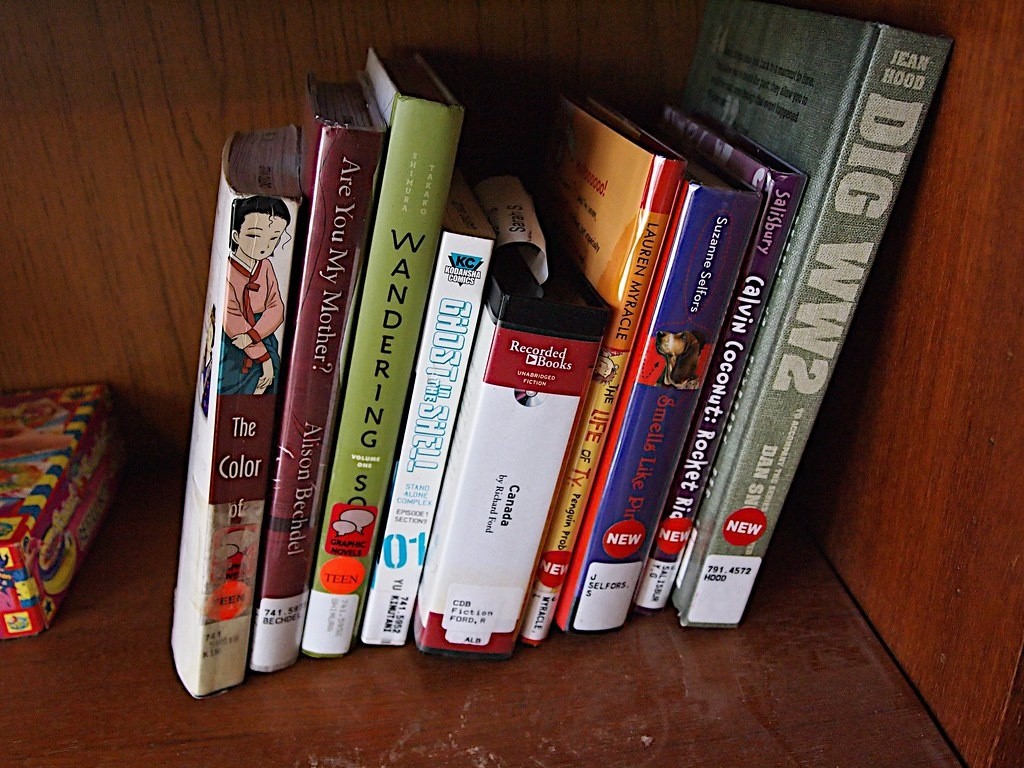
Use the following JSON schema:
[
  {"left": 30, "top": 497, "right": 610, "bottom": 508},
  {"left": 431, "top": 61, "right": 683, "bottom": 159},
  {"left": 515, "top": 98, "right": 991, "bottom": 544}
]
[{"left": 656, "top": 330, "right": 706, "bottom": 390}]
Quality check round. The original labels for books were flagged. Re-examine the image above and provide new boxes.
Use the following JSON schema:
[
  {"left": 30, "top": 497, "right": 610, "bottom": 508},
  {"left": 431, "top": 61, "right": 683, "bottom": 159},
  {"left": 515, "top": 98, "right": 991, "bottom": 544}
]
[
  {"left": 520, "top": 79, "right": 808, "bottom": 649},
  {"left": 248, "top": 66, "right": 388, "bottom": 673},
  {"left": 300, "top": 45, "right": 466, "bottom": 658},
  {"left": 170, "top": 123, "right": 305, "bottom": 699},
  {"left": 410, "top": 157, "right": 613, "bottom": 661},
  {"left": 360, "top": 179, "right": 497, "bottom": 646},
  {"left": 670, "top": 1, "right": 955, "bottom": 628}
]
